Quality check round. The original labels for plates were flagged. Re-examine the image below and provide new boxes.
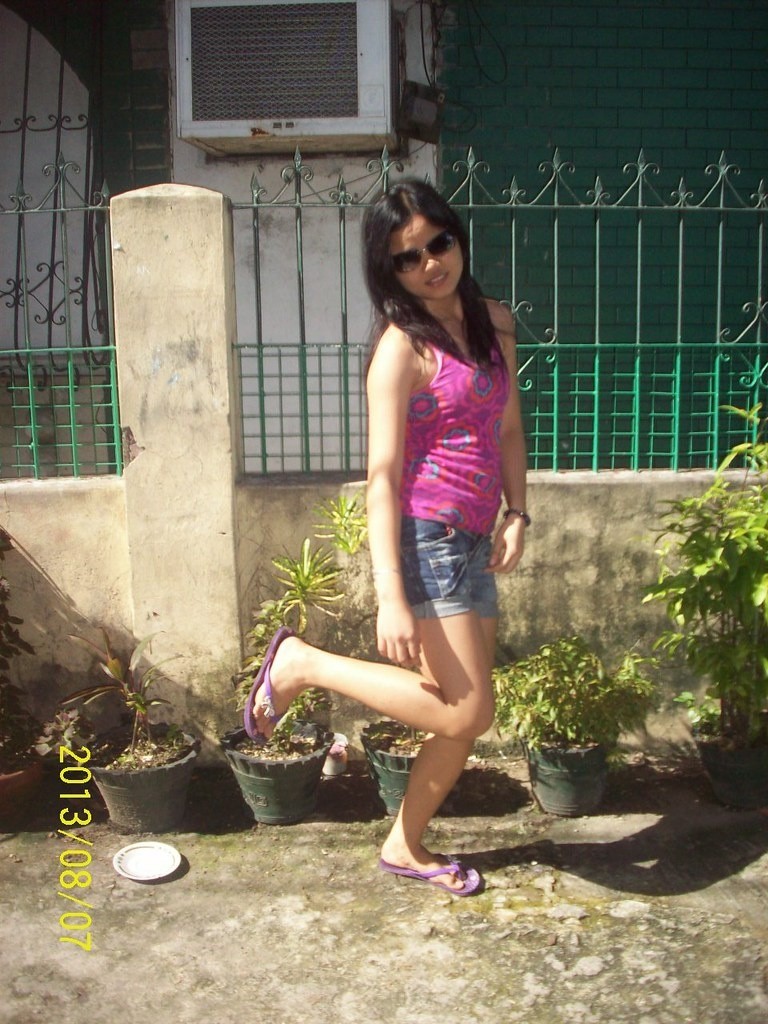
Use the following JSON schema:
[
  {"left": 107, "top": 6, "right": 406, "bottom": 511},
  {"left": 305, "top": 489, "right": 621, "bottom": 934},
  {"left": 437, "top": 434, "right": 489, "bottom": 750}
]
[{"left": 113, "top": 840, "right": 182, "bottom": 881}]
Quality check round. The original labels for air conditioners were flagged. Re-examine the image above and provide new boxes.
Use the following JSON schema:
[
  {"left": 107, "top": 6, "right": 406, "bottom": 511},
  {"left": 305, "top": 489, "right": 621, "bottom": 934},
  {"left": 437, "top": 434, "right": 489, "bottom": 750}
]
[{"left": 172, "top": 0, "right": 401, "bottom": 155}]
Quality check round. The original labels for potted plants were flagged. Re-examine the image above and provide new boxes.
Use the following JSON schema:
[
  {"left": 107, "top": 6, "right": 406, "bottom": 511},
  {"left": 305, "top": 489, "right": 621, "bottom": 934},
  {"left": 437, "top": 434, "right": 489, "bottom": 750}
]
[
  {"left": 0, "top": 529, "right": 44, "bottom": 824},
  {"left": 31, "top": 705, "right": 115, "bottom": 812},
  {"left": 490, "top": 636, "right": 662, "bottom": 819},
  {"left": 314, "top": 489, "right": 418, "bottom": 817},
  {"left": 639, "top": 403, "right": 768, "bottom": 811},
  {"left": 218, "top": 538, "right": 344, "bottom": 826},
  {"left": 68, "top": 624, "right": 201, "bottom": 834}
]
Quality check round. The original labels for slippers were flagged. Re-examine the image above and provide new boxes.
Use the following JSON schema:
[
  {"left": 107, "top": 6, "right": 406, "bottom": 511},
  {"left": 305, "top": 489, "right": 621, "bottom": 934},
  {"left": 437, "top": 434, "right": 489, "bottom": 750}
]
[
  {"left": 244, "top": 626, "right": 297, "bottom": 744},
  {"left": 379, "top": 853, "right": 480, "bottom": 896}
]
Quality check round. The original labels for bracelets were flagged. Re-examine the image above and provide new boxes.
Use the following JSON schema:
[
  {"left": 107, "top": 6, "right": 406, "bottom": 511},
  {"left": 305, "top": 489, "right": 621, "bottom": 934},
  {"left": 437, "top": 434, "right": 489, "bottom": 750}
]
[{"left": 503, "top": 508, "right": 531, "bottom": 526}]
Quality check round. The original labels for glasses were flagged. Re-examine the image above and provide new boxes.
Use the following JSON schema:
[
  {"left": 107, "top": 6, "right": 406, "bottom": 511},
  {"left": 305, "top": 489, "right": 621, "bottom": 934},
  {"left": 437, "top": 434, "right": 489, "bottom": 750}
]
[{"left": 389, "top": 228, "right": 456, "bottom": 273}]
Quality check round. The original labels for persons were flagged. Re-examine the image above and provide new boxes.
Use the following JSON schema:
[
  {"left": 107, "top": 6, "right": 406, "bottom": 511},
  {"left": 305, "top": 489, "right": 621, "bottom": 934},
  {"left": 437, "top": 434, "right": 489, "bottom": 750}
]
[{"left": 244, "top": 178, "right": 533, "bottom": 895}]
